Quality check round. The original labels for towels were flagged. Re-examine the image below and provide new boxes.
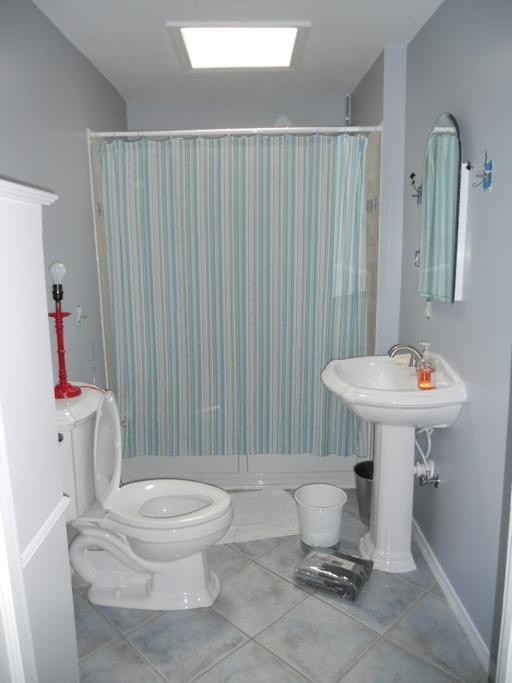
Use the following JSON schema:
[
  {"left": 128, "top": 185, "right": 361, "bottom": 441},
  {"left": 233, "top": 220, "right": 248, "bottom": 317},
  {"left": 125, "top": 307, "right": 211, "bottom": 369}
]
[{"left": 214, "top": 488, "right": 301, "bottom": 546}]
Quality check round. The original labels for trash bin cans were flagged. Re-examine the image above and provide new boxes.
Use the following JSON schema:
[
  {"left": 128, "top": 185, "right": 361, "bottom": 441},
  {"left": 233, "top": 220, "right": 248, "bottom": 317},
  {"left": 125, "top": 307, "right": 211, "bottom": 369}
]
[{"left": 354, "top": 460, "right": 373, "bottom": 526}]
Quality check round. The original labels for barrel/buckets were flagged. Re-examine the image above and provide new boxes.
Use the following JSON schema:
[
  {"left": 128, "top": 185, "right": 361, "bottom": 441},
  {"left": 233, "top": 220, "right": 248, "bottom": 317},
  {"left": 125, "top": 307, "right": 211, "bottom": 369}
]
[{"left": 352, "top": 461, "right": 374, "bottom": 528}]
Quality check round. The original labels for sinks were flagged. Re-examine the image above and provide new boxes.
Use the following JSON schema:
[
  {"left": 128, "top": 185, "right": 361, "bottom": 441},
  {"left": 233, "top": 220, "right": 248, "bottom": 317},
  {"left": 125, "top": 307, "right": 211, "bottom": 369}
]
[{"left": 321, "top": 351, "right": 469, "bottom": 428}]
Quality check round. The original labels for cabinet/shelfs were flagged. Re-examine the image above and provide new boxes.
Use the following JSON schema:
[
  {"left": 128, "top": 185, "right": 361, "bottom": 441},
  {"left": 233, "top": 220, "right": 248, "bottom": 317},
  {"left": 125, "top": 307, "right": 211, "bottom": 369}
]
[{"left": 1, "top": 175, "right": 81, "bottom": 683}]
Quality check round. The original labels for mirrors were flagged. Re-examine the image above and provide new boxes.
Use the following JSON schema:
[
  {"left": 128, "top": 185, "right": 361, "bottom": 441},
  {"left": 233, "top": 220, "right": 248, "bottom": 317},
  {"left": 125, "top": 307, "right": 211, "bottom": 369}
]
[{"left": 419, "top": 112, "right": 462, "bottom": 306}]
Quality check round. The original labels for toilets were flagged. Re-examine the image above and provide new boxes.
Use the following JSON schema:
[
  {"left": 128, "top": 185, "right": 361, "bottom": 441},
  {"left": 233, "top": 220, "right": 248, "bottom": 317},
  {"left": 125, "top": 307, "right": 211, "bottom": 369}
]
[{"left": 57, "top": 381, "right": 235, "bottom": 610}]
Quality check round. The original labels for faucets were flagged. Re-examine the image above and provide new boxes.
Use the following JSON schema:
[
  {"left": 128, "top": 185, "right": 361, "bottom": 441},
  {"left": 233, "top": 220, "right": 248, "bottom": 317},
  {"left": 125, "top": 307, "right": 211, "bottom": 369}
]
[{"left": 387, "top": 344, "right": 426, "bottom": 372}]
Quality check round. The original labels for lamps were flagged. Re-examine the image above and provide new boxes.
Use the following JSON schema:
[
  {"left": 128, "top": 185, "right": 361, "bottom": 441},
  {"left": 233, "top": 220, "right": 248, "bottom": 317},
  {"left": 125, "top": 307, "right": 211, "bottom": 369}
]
[{"left": 46, "top": 262, "right": 81, "bottom": 398}]
